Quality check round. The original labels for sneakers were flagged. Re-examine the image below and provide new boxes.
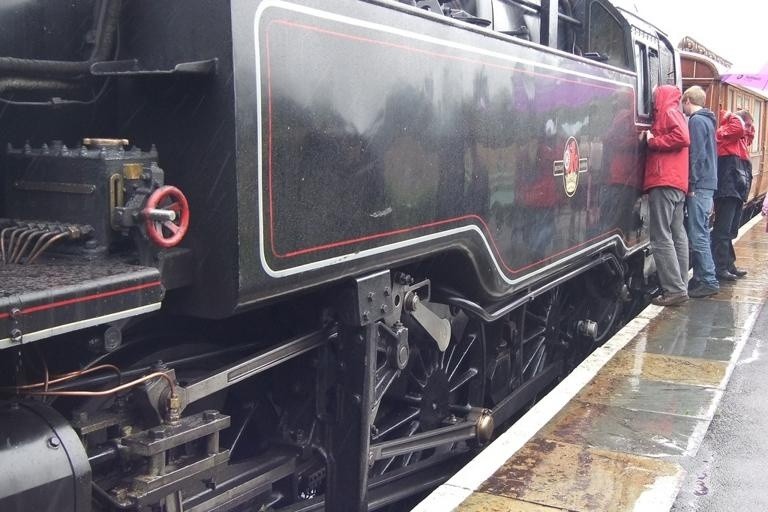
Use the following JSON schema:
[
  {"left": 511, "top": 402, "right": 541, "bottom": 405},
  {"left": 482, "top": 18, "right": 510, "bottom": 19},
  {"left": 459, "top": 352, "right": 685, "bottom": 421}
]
[{"left": 651, "top": 270, "right": 747, "bottom": 306}]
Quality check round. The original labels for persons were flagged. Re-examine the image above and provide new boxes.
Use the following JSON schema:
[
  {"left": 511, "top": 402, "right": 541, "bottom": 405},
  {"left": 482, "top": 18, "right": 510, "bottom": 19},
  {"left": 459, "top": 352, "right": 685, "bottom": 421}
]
[
  {"left": 512, "top": 86, "right": 644, "bottom": 269},
  {"left": 644, "top": 84, "right": 755, "bottom": 307},
  {"left": 761, "top": 192, "right": 768, "bottom": 233}
]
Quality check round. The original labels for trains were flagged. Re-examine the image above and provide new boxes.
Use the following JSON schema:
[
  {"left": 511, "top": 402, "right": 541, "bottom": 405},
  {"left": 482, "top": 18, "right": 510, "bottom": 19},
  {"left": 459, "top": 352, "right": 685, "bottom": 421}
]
[{"left": 0, "top": 0, "right": 768, "bottom": 512}]
